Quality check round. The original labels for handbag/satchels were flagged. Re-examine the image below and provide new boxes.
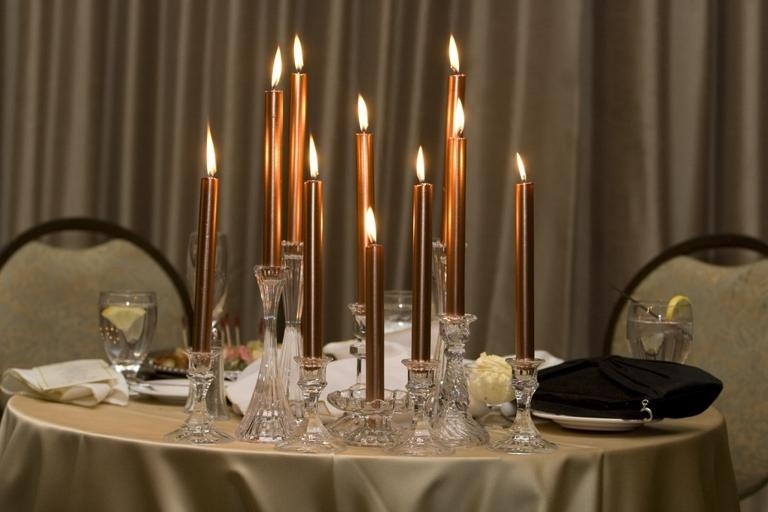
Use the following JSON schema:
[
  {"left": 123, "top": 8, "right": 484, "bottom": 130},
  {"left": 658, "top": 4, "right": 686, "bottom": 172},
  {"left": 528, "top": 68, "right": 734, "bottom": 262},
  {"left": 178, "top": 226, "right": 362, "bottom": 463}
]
[{"left": 529, "top": 354, "right": 724, "bottom": 421}]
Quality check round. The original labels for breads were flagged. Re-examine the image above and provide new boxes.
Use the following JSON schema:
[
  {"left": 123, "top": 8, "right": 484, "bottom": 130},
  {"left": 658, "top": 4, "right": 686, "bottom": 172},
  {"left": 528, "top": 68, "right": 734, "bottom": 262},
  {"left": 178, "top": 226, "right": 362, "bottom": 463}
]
[{"left": 152, "top": 350, "right": 190, "bottom": 369}]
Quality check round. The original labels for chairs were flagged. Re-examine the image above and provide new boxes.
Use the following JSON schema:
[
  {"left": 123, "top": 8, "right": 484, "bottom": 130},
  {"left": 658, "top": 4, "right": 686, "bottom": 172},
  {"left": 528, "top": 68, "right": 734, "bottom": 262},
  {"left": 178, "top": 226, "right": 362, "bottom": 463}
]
[
  {"left": 604, "top": 233, "right": 768, "bottom": 501},
  {"left": 0, "top": 217, "right": 192, "bottom": 405}
]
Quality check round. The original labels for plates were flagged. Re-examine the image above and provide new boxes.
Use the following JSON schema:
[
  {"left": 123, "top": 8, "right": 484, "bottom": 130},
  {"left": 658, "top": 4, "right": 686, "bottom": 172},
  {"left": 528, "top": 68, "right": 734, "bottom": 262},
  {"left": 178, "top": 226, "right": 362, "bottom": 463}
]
[
  {"left": 131, "top": 378, "right": 188, "bottom": 405},
  {"left": 532, "top": 411, "right": 666, "bottom": 432}
]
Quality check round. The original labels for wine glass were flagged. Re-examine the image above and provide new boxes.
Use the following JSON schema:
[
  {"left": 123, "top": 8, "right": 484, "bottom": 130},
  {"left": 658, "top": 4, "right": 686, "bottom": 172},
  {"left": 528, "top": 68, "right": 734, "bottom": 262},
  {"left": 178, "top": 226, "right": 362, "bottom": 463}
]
[
  {"left": 469, "top": 362, "right": 515, "bottom": 429},
  {"left": 97, "top": 292, "right": 158, "bottom": 394},
  {"left": 186, "top": 232, "right": 226, "bottom": 343}
]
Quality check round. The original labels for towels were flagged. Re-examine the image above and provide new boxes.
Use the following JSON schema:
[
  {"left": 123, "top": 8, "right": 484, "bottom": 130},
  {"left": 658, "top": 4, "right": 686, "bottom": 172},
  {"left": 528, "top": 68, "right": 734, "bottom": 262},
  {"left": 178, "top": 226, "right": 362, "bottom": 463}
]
[{"left": 0, "top": 359, "right": 130, "bottom": 407}]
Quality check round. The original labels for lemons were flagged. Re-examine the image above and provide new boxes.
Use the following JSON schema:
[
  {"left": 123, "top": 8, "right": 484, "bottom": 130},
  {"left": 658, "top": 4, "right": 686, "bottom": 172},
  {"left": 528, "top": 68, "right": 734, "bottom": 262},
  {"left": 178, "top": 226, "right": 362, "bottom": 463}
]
[
  {"left": 104, "top": 306, "right": 148, "bottom": 332},
  {"left": 665, "top": 295, "right": 694, "bottom": 323}
]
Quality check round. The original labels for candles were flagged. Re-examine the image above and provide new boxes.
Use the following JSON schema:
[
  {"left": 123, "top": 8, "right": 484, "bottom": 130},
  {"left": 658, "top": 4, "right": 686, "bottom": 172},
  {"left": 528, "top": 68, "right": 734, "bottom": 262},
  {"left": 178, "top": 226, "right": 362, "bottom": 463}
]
[
  {"left": 444, "top": 96, "right": 470, "bottom": 319},
  {"left": 441, "top": 36, "right": 469, "bottom": 247},
  {"left": 302, "top": 132, "right": 324, "bottom": 370},
  {"left": 354, "top": 92, "right": 374, "bottom": 306},
  {"left": 363, "top": 203, "right": 388, "bottom": 410},
  {"left": 410, "top": 143, "right": 433, "bottom": 382},
  {"left": 263, "top": 44, "right": 285, "bottom": 278},
  {"left": 192, "top": 122, "right": 219, "bottom": 354},
  {"left": 284, "top": 36, "right": 308, "bottom": 254},
  {"left": 515, "top": 149, "right": 533, "bottom": 374}
]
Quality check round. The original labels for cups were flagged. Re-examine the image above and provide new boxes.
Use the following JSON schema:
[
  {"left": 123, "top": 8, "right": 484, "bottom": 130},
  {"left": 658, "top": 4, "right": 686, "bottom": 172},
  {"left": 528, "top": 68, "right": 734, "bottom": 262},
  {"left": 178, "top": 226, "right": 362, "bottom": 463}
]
[
  {"left": 382, "top": 290, "right": 419, "bottom": 338},
  {"left": 626, "top": 300, "right": 694, "bottom": 364}
]
